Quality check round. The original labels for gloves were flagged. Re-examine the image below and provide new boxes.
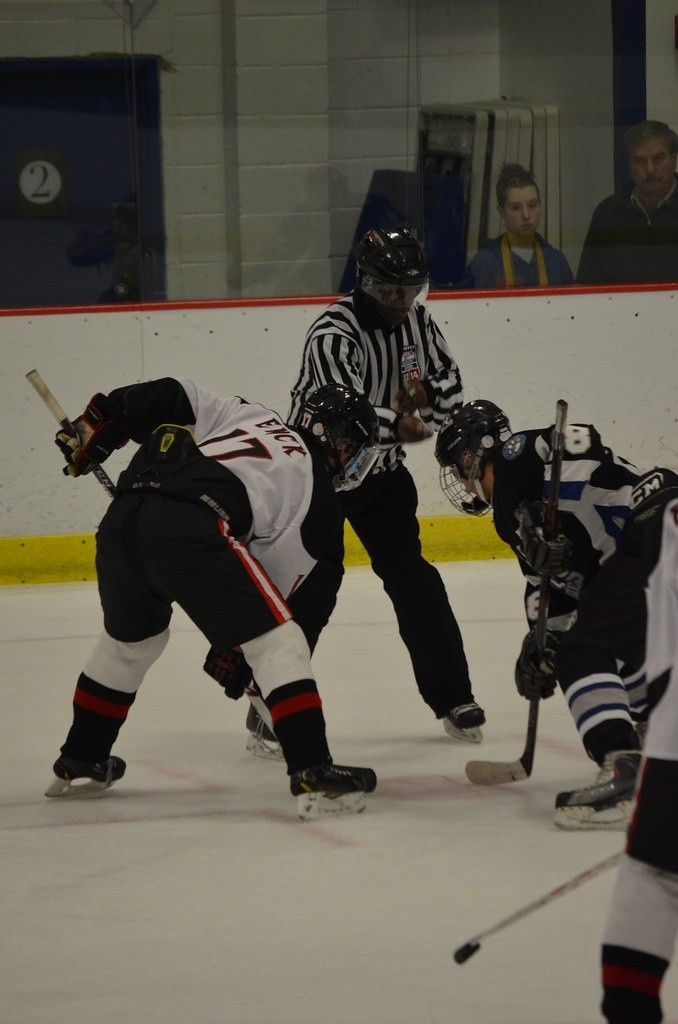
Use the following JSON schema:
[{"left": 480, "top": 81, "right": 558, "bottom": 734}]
[
  {"left": 203, "top": 643, "right": 253, "bottom": 700},
  {"left": 54, "top": 393, "right": 130, "bottom": 478},
  {"left": 514, "top": 627, "right": 560, "bottom": 701}
]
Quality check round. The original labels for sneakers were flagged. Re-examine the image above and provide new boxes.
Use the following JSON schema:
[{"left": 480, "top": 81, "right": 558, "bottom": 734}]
[
  {"left": 245, "top": 677, "right": 287, "bottom": 761},
  {"left": 45, "top": 753, "right": 127, "bottom": 797},
  {"left": 553, "top": 732, "right": 643, "bottom": 831},
  {"left": 442, "top": 702, "right": 486, "bottom": 743},
  {"left": 290, "top": 757, "right": 378, "bottom": 819}
]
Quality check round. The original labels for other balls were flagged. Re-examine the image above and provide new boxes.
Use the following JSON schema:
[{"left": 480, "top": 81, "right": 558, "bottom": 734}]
[{"left": 454, "top": 942, "right": 480, "bottom": 965}]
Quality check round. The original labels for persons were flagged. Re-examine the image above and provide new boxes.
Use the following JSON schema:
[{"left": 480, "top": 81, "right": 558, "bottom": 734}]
[
  {"left": 573, "top": 118, "right": 678, "bottom": 286},
  {"left": 44, "top": 376, "right": 380, "bottom": 821},
  {"left": 432, "top": 395, "right": 678, "bottom": 832},
  {"left": 578, "top": 502, "right": 678, "bottom": 1023},
  {"left": 244, "top": 226, "right": 487, "bottom": 762},
  {"left": 460, "top": 162, "right": 574, "bottom": 291}
]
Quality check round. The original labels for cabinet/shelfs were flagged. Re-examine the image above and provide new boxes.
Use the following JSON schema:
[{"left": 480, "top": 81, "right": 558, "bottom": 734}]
[{"left": 0, "top": 52, "right": 174, "bottom": 308}]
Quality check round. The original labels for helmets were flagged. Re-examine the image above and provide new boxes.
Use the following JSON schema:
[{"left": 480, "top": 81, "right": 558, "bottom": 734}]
[
  {"left": 304, "top": 382, "right": 382, "bottom": 446},
  {"left": 353, "top": 223, "right": 430, "bottom": 286},
  {"left": 432, "top": 398, "right": 513, "bottom": 517}
]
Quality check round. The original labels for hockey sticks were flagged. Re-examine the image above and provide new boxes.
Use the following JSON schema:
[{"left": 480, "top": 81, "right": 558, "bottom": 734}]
[
  {"left": 464, "top": 396, "right": 569, "bottom": 788},
  {"left": 20, "top": 363, "right": 380, "bottom": 799},
  {"left": 451, "top": 849, "right": 625, "bottom": 966}
]
